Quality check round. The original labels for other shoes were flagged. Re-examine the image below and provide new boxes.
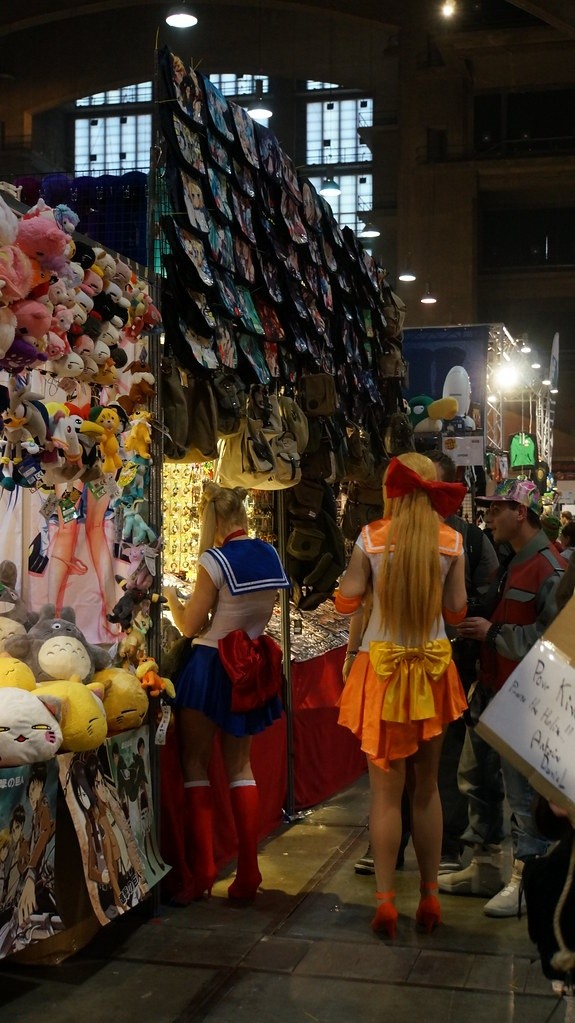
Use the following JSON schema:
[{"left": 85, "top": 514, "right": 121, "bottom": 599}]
[
  {"left": 440, "top": 851, "right": 462, "bottom": 874},
  {"left": 354, "top": 845, "right": 404, "bottom": 873}
]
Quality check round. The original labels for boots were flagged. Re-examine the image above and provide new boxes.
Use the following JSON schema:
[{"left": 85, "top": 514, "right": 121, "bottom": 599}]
[
  {"left": 174, "top": 780, "right": 218, "bottom": 903},
  {"left": 228, "top": 779, "right": 261, "bottom": 904}
]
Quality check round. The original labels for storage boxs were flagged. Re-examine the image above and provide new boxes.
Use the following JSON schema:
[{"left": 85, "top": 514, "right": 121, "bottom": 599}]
[{"left": 473, "top": 596, "right": 575, "bottom": 818}]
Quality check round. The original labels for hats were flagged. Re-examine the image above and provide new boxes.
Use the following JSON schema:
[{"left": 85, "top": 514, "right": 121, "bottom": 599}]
[
  {"left": 474, "top": 478, "right": 542, "bottom": 518},
  {"left": 541, "top": 516, "right": 562, "bottom": 541}
]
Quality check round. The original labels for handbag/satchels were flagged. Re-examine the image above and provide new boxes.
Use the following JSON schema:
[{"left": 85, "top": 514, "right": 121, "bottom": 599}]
[{"left": 158, "top": 267, "right": 415, "bottom": 611}]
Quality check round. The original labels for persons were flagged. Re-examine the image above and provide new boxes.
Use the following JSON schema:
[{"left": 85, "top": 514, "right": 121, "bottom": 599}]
[
  {"left": 438, "top": 479, "right": 575, "bottom": 1023},
  {"left": 342, "top": 452, "right": 499, "bottom": 876},
  {"left": 336, "top": 452, "right": 467, "bottom": 944},
  {"left": 165, "top": 484, "right": 288, "bottom": 904}
]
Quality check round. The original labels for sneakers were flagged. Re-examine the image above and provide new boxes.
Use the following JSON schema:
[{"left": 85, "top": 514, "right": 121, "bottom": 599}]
[
  {"left": 439, "top": 855, "right": 502, "bottom": 897},
  {"left": 483, "top": 859, "right": 527, "bottom": 918}
]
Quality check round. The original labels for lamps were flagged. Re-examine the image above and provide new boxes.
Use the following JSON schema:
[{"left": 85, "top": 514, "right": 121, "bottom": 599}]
[
  {"left": 420, "top": 282, "right": 437, "bottom": 304},
  {"left": 541, "top": 368, "right": 552, "bottom": 385},
  {"left": 359, "top": 209, "right": 380, "bottom": 238},
  {"left": 165, "top": 0, "right": 198, "bottom": 29},
  {"left": 319, "top": 165, "right": 341, "bottom": 196},
  {"left": 398, "top": 251, "right": 417, "bottom": 282},
  {"left": 247, "top": 79, "right": 274, "bottom": 120},
  {"left": 550, "top": 382, "right": 559, "bottom": 394},
  {"left": 529, "top": 350, "right": 542, "bottom": 368},
  {"left": 516, "top": 339, "right": 532, "bottom": 354}
]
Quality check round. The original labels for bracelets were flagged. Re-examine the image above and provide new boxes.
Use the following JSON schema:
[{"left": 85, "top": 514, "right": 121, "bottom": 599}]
[{"left": 344, "top": 651, "right": 357, "bottom": 660}]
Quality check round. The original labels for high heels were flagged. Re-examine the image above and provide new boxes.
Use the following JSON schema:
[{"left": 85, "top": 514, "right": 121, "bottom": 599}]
[
  {"left": 416, "top": 894, "right": 441, "bottom": 938},
  {"left": 370, "top": 902, "right": 399, "bottom": 945}
]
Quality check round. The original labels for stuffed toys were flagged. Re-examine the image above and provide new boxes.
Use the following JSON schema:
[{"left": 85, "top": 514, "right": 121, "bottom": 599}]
[
  {"left": 0, "top": 196, "right": 164, "bottom": 591},
  {"left": 0, "top": 561, "right": 175, "bottom": 767},
  {"left": 410, "top": 395, "right": 458, "bottom": 432}
]
